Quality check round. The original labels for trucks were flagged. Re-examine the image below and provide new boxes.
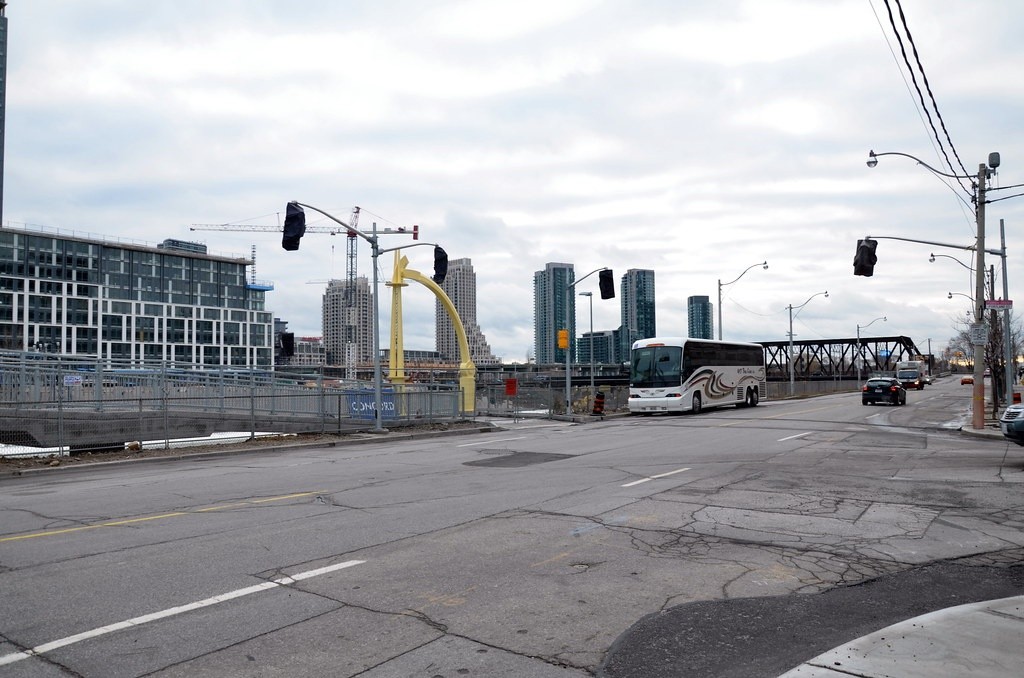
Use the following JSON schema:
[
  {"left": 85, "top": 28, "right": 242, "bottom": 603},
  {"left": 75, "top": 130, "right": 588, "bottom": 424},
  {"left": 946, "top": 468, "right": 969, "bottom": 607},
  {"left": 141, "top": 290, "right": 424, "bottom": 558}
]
[{"left": 894, "top": 361, "right": 925, "bottom": 391}]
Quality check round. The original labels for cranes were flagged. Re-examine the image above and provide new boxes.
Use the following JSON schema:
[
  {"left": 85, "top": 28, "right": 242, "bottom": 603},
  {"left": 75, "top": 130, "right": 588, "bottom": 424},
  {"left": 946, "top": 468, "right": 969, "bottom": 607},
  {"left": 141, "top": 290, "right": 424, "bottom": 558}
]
[{"left": 189, "top": 206, "right": 420, "bottom": 380}]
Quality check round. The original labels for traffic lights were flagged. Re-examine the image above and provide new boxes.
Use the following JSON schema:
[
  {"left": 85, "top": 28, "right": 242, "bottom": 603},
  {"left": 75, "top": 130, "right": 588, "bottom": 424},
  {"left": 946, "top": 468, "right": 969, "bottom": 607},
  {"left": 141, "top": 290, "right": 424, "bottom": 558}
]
[{"left": 599, "top": 269, "right": 615, "bottom": 300}]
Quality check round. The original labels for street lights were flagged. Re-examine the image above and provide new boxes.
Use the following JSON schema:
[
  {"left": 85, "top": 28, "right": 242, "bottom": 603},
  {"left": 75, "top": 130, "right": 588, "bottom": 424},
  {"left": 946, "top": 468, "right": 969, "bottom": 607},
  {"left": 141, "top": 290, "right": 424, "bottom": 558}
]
[
  {"left": 32, "top": 340, "right": 60, "bottom": 354},
  {"left": 718, "top": 260, "right": 769, "bottom": 341},
  {"left": 857, "top": 317, "right": 887, "bottom": 389},
  {"left": 865, "top": 150, "right": 998, "bottom": 430},
  {"left": 578, "top": 291, "right": 594, "bottom": 409},
  {"left": 788, "top": 290, "right": 829, "bottom": 396}
]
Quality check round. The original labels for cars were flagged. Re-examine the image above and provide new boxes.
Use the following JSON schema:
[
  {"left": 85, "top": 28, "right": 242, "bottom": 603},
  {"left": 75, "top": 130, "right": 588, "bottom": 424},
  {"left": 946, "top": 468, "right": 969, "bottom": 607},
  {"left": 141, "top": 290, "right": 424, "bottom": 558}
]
[
  {"left": 925, "top": 375, "right": 931, "bottom": 385},
  {"left": 984, "top": 368, "right": 991, "bottom": 378},
  {"left": 961, "top": 376, "right": 974, "bottom": 385}
]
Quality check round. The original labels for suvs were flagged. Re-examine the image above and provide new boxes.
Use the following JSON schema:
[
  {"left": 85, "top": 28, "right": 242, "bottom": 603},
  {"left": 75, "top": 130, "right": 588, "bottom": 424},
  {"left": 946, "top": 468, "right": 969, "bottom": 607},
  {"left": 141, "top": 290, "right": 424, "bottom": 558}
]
[
  {"left": 861, "top": 378, "right": 907, "bottom": 406},
  {"left": 1000, "top": 403, "right": 1024, "bottom": 443}
]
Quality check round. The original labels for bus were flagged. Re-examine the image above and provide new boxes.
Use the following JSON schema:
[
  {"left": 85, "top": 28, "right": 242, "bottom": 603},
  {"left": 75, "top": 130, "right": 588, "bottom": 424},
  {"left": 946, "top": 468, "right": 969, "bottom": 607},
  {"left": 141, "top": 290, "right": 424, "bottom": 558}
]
[{"left": 627, "top": 336, "right": 768, "bottom": 414}]
